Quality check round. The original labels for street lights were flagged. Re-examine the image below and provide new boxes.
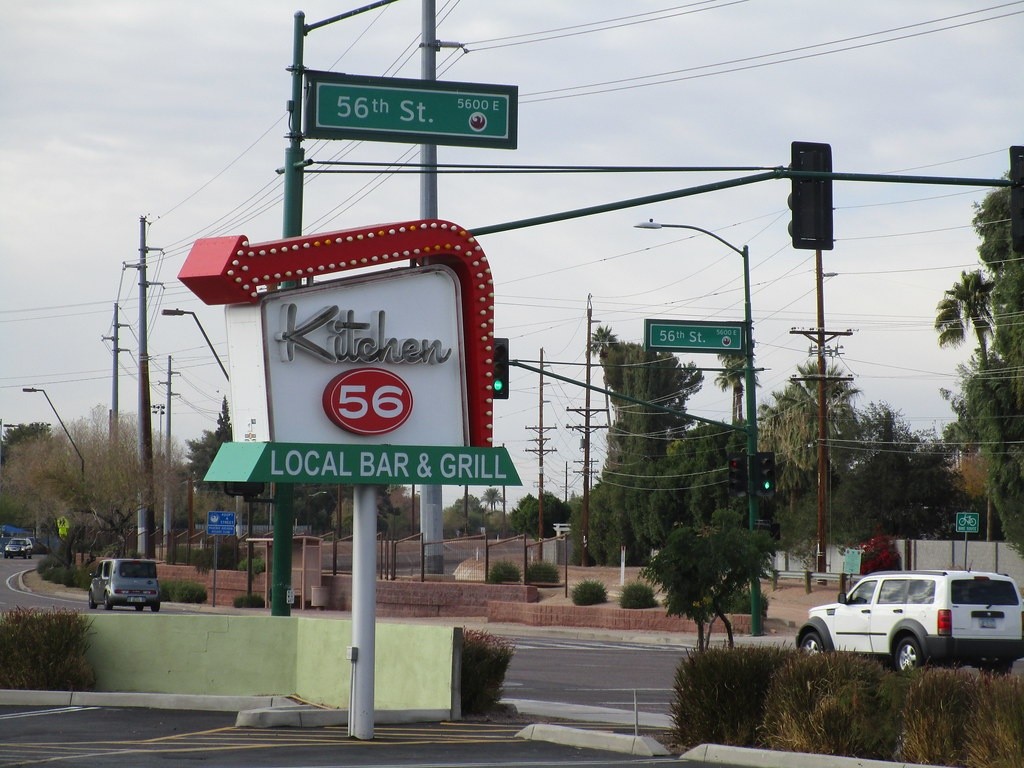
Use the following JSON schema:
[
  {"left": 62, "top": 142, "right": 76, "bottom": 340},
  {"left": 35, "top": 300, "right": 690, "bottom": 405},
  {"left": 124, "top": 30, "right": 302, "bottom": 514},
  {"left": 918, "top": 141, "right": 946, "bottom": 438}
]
[
  {"left": 22, "top": 387, "right": 85, "bottom": 481},
  {"left": 159, "top": 309, "right": 232, "bottom": 383},
  {"left": 634, "top": 218, "right": 763, "bottom": 637}
]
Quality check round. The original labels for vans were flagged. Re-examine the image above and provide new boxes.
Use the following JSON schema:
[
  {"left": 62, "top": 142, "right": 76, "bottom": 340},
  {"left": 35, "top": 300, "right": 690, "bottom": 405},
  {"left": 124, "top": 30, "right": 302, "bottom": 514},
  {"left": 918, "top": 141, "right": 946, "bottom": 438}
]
[{"left": 88, "top": 558, "right": 161, "bottom": 613}]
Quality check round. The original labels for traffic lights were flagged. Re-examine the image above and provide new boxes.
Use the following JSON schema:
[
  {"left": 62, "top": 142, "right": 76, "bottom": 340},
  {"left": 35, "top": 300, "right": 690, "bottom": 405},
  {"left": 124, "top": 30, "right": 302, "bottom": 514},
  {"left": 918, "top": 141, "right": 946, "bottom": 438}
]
[
  {"left": 727, "top": 450, "right": 748, "bottom": 498},
  {"left": 754, "top": 451, "right": 776, "bottom": 494}
]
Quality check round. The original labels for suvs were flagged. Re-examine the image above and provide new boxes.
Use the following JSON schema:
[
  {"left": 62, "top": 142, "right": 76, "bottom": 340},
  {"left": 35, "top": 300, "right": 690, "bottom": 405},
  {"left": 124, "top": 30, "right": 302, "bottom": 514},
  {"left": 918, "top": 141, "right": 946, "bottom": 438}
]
[
  {"left": 795, "top": 569, "right": 1024, "bottom": 679},
  {"left": 4, "top": 537, "right": 33, "bottom": 559}
]
